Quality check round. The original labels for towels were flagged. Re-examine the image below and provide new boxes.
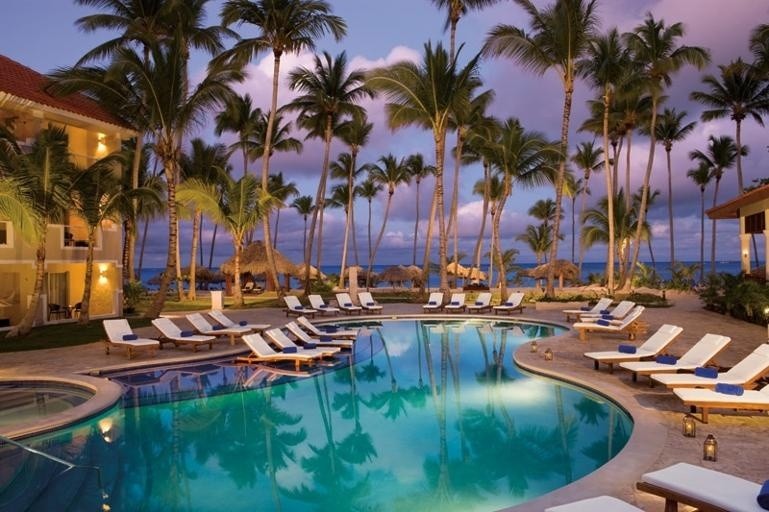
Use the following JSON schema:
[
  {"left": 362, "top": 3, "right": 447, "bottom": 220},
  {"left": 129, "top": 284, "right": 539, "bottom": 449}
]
[{"left": 758, "top": 478, "right": 769, "bottom": 512}]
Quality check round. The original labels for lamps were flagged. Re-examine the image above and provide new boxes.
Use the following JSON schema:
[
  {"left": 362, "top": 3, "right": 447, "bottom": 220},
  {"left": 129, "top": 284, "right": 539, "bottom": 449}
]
[
  {"left": 96, "top": 132, "right": 105, "bottom": 150},
  {"left": 530, "top": 341, "right": 538, "bottom": 353},
  {"left": 703, "top": 434, "right": 718, "bottom": 462},
  {"left": 681, "top": 412, "right": 696, "bottom": 437},
  {"left": 544, "top": 347, "right": 554, "bottom": 360},
  {"left": 97, "top": 263, "right": 109, "bottom": 286}
]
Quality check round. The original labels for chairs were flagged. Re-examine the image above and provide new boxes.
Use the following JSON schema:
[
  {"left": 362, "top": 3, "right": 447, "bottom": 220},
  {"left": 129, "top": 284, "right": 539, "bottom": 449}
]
[
  {"left": 633, "top": 461, "right": 768, "bottom": 512},
  {"left": 74, "top": 303, "right": 81, "bottom": 318},
  {"left": 546, "top": 495, "right": 644, "bottom": 512},
  {"left": 48, "top": 303, "right": 67, "bottom": 321},
  {"left": 102, "top": 289, "right": 383, "bottom": 371},
  {"left": 108, "top": 342, "right": 354, "bottom": 387},
  {"left": 421, "top": 292, "right": 650, "bottom": 341},
  {"left": 582, "top": 321, "right": 769, "bottom": 424}
]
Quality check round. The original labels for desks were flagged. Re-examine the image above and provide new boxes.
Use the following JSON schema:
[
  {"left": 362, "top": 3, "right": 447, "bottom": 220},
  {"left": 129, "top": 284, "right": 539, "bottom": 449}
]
[{"left": 63, "top": 305, "right": 76, "bottom": 319}]
